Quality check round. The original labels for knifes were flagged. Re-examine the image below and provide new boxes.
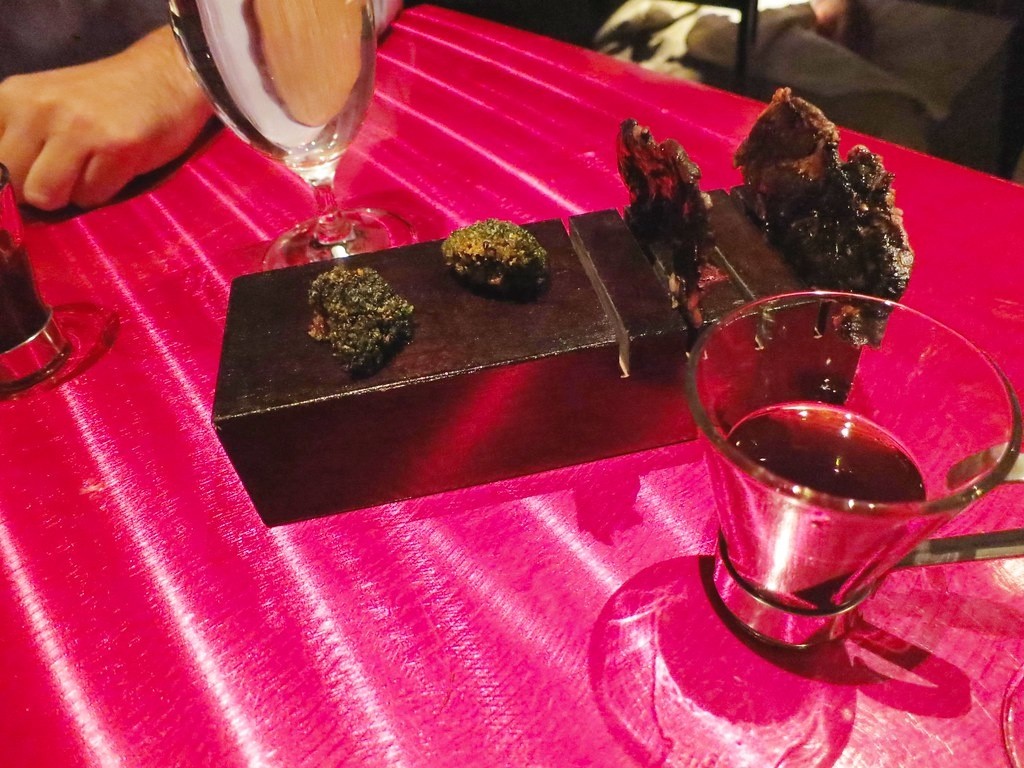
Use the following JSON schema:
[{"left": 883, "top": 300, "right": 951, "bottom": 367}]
[{"left": 946, "top": 442, "right": 1024, "bottom": 489}]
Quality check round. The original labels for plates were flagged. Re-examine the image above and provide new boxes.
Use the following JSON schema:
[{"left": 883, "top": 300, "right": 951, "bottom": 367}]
[{"left": 1001, "top": 664, "right": 1024, "bottom": 768}]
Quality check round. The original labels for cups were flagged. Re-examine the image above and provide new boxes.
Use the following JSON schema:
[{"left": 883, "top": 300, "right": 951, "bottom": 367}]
[
  {"left": 1, "top": 164, "right": 73, "bottom": 395},
  {"left": 684, "top": 291, "right": 1024, "bottom": 651}
]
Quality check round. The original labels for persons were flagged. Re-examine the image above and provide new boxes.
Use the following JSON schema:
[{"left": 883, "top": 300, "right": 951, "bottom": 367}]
[
  {"left": 675, "top": 0, "right": 1024, "bottom": 184},
  {"left": 0, "top": 0, "right": 402, "bottom": 213}
]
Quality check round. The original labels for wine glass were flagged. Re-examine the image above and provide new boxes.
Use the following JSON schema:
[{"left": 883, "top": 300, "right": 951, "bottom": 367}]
[{"left": 169, "top": 0, "right": 417, "bottom": 273}]
